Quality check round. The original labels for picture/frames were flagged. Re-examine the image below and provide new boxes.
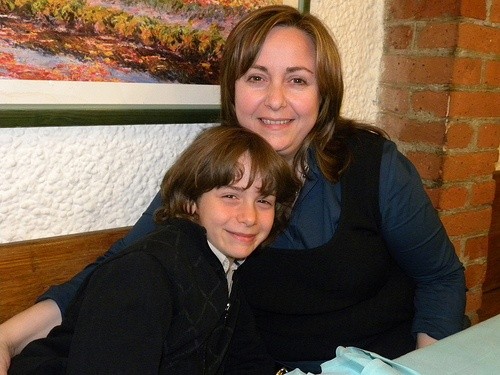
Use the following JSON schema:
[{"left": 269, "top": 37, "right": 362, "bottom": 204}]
[{"left": 0, "top": 0, "right": 311, "bottom": 128}]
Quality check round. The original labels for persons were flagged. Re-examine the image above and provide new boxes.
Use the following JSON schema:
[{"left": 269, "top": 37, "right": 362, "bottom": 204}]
[
  {"left": 0, "top": 4, "right": 466, "bottom": 375},
  {"left": 6, "top": 127, "right": 299, "bottom": 375}
]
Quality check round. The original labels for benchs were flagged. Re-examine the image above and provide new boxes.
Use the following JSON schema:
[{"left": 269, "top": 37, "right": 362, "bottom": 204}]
[{"left": 0, "top": 225, "right": 135, "bottom": 324}]
